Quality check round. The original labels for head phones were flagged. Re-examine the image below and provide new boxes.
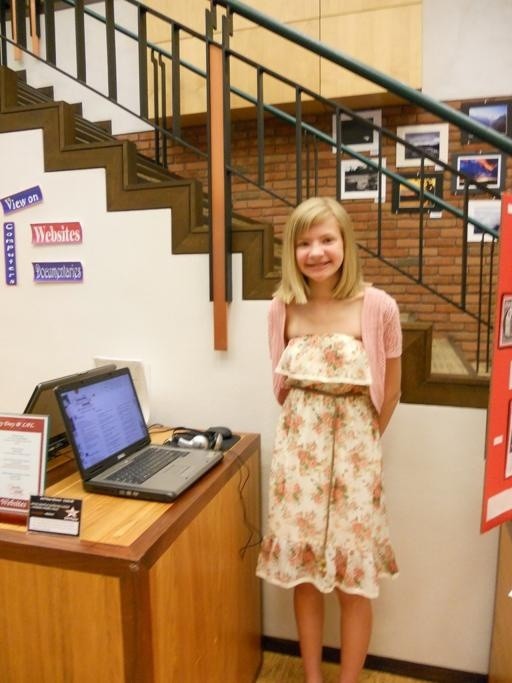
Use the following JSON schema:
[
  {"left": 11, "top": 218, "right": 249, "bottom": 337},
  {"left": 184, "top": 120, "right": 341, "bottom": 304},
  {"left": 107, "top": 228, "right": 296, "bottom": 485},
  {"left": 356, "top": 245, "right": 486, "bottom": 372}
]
[{"left": 171, "top": 426, "right": 224, "bottom": 450}]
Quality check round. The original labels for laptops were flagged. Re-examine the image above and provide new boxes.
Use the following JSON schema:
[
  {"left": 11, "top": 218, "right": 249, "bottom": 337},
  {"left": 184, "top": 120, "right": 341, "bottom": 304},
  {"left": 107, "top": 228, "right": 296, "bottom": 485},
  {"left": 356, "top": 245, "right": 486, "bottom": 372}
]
[{"left": 53, "top": 366, "right": 224, "bottom": 502}]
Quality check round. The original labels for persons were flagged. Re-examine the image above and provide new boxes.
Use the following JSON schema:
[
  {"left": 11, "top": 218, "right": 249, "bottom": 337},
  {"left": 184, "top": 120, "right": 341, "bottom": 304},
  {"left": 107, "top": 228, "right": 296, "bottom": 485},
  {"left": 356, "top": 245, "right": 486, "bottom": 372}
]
[{"left": 255, "top": 196, "right": 402, "bottom": 683}]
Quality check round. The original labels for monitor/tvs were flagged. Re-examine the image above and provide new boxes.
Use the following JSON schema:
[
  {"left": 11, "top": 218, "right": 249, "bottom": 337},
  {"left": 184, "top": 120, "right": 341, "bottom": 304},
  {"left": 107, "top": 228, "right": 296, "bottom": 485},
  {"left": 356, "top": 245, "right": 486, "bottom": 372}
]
[{"left": 22, "top": 363, "right": 117, "bottom": 454}]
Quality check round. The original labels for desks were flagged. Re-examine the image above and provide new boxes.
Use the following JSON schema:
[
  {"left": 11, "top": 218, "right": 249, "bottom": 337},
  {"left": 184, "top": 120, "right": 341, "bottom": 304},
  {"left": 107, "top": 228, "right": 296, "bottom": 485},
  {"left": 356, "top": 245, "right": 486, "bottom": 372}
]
[{"left": 0, "top": 425, "right": 262, "bottom": 683}]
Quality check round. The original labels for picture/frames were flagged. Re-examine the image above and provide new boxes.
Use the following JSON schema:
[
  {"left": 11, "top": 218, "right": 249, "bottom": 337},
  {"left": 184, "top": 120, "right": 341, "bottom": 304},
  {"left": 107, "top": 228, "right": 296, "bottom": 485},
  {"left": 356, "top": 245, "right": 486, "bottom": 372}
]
[{"left": 331, "top": 100, "right": 512, "bottom": 242}]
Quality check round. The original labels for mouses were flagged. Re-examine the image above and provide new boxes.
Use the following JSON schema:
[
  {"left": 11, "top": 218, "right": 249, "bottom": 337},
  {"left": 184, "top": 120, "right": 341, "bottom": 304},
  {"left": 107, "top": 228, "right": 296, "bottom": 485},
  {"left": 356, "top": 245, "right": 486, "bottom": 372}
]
[{"left": 207, "top": 426, "right": 232, "bottom": 439}]
